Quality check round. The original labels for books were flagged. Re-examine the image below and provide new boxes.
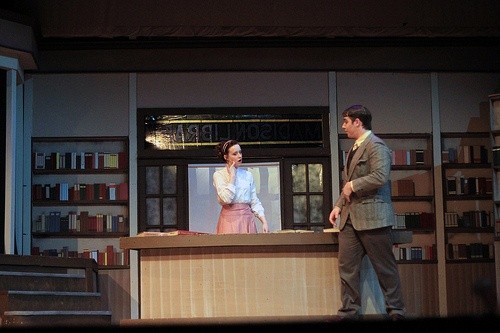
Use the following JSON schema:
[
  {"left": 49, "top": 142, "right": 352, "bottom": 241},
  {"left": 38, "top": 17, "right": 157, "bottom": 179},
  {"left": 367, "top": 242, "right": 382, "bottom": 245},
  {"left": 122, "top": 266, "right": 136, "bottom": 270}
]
[
  {"left": 447, "top": 171, "right": 493, "bottom": 195},
  {"left": 392, "top": 244, "right": 437, "bottom": 261},
  {"left": 391, "top": 149, "right": 424, "bottom": 166},
  {"left": 446, "top": 243, "right": 493, "bottom": 259},
  {"left": 492, "top": 146, "right": 500, "bottom": 166},
  {"left": 137, "top": 231, "right": 211, "bottom": 237},
  {"left": 392, "top": 180, "right": 415, "bottom": 196},
  {"left": 445, "top": 210, "right": 495, "bottom": 227},
  {"left": 442, "top": 145, "right": 488, "bottom": 163},
  {"left": 32, "top": 245, "right": 128, "bottom": 265},
  {"left": 33, "top": 182, "right": 128, "bottom": 201},
  {"left": 392, "top": 212, "right": 435, "bottom": 229},
  {"left": 33, "top": 152, "right": 125, "bottom": 169},
  {"left": 32, "top": 211, "right": 129, "bottom": 233}
]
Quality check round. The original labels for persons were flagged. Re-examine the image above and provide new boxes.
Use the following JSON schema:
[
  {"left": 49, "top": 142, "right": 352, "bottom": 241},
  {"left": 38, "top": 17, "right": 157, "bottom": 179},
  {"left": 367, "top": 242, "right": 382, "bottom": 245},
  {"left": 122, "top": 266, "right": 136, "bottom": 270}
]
[
  {"left": 213, "top": 140, "right": 269, "bottom": 234},
  {"left": 329, "top": 105, "right": 407, "bottom": 322}
]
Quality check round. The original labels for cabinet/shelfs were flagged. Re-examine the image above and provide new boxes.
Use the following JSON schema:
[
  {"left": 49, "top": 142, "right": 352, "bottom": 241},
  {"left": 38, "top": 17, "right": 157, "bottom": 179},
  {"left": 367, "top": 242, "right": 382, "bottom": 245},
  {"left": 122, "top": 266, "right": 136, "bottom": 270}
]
[
  {"left": 440, "top": 131, "right": 495, "bottom": 264},
  {"left": 30, "top": 136, "right": 130, "bottom": 269},
  {"left": 338, "top": 133, "right": 438, "bottom": 265}
]
